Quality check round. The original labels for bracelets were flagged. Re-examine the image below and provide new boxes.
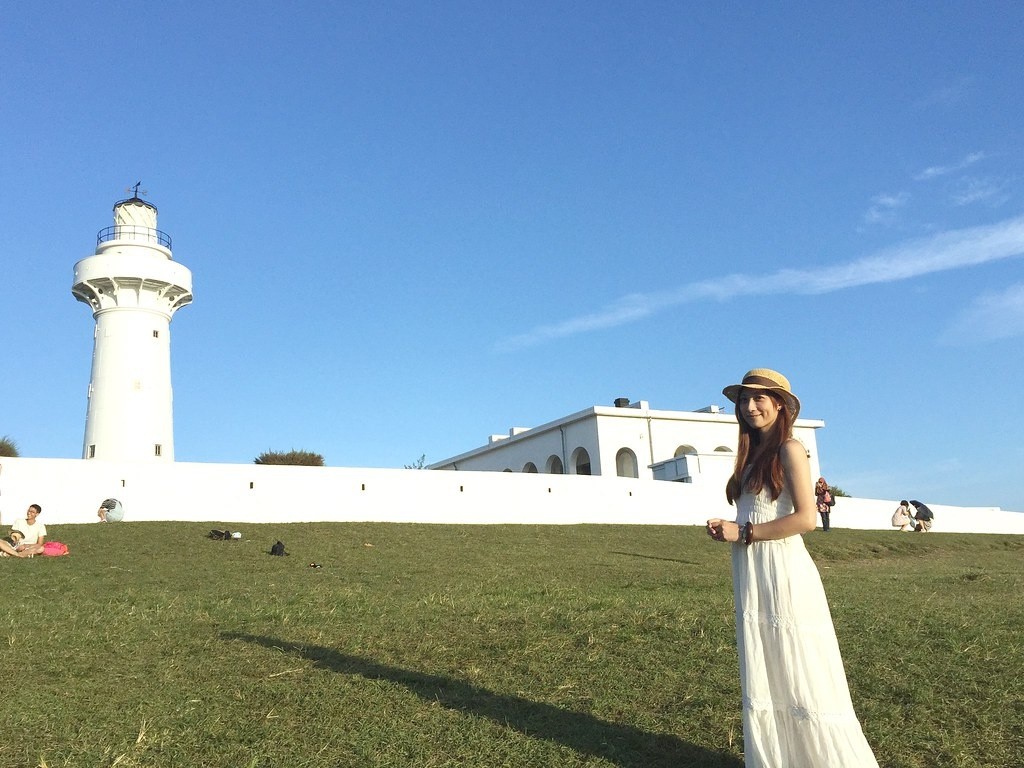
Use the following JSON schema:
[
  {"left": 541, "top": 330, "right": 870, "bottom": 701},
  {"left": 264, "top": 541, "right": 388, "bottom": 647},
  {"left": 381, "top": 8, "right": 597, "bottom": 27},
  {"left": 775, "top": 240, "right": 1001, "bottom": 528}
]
[{"left": 739, "top": 521, "right": 753, "bottom": 545}]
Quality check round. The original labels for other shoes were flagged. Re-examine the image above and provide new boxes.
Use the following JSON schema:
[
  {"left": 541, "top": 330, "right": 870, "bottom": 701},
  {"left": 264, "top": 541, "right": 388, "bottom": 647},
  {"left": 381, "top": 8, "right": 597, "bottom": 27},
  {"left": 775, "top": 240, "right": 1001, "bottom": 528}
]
[
  {"left": 920, "top": 529, "right": 926, "bottom": 533},
  {"left": 900, "top": 528, "right": 907, "bottom": 531}
]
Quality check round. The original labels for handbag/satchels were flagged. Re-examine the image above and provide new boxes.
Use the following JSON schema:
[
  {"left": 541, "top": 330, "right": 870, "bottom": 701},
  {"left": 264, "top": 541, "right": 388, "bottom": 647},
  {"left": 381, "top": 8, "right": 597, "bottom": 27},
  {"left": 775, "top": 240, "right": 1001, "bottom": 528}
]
[{"left": 824, "top": 490, "right": 835, "bottom": 506}]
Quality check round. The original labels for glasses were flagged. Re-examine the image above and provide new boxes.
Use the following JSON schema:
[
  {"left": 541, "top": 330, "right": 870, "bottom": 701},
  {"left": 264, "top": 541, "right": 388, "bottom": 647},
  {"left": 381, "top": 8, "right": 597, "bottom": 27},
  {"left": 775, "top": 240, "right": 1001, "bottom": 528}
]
[{"left": 819, "top": 482, "right": 823, "bottom": 484}]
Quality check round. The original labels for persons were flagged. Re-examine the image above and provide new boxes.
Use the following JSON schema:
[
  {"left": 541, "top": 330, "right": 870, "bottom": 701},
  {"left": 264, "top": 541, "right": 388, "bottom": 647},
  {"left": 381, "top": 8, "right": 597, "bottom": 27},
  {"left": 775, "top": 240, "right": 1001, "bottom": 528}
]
[
  {"left": 98, "top": 499, "right": 124, "bottom": 523},
  {"left": 706, "top": 368, "right": 880, "bottom": 768},
  {"left": 909, "top": 507, "right": 931, "bottom": 532},
  {"left": 0, "top": 504, "right": 47, "bottom": 558},
  {"left": 815, "top": 478, "right": 830, "bottom": 532},
  {"left": 892, "top": 500, "right": 911, "bottom": 532}
]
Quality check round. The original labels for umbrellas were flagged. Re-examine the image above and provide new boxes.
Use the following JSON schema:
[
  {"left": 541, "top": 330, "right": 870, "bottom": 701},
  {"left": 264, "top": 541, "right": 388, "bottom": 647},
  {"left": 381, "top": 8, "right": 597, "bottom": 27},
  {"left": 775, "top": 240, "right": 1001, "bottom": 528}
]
[{"left": 910, "top": 500, "right": 934, "bottom": 519}]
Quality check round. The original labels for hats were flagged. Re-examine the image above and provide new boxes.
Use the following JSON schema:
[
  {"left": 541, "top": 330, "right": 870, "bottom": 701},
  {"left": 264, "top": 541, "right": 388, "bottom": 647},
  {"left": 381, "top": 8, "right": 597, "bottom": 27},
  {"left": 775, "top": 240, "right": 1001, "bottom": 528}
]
[{"left": 722, "top": 369, "right": 800, "bottom": 426}]
